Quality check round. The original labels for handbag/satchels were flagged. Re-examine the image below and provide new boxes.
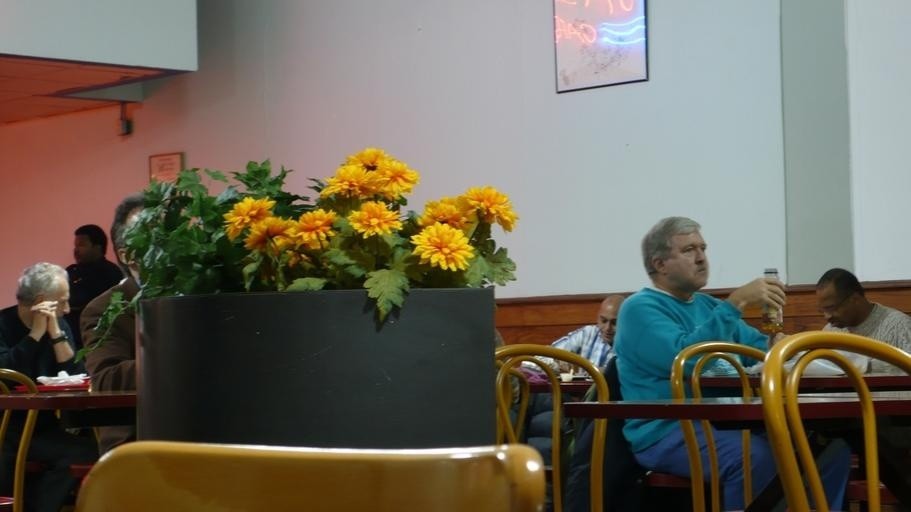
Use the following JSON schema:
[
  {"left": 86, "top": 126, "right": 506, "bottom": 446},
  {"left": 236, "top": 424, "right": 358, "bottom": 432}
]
[{"left": 50, "top": 329, "right": 68, "bottom": 345}]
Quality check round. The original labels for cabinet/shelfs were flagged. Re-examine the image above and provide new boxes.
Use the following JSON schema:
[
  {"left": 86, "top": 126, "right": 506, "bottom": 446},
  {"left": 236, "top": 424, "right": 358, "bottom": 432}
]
[{"left": 762, "top": 270, "right": 782, "bottom": 333}]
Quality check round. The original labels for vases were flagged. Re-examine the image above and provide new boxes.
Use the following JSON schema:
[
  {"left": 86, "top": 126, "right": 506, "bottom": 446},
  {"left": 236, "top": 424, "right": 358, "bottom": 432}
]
[{"left": 103, "top": 149, "right": 518, "bottom": 287}]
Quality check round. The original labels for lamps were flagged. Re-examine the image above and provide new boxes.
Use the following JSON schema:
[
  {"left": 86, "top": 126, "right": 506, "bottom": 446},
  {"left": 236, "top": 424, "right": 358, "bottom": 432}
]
[
  {"left": 494, "top": 343, "right": 609, "bottom": 512},
  {"left": 761, "top": 330, "right": 910, "bottom": 512},
  {"left": 496, "top": 360, "right": 529, "bottom": 444},
  {"left": 671, "top": 340, "right": 794, "bottom": 512},
  {"left": 0, "top": 368, "right": 39, "bottom": 512}
]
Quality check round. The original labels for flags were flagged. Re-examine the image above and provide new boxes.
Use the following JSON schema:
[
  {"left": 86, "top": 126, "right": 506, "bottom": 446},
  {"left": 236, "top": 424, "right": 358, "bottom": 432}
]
[{"left": 136, "top": 290, "right": 497, "bottom": 447}]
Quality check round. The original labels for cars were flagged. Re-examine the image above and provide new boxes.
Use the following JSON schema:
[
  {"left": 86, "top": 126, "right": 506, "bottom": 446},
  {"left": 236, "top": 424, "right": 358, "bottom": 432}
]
[{"left": 561, "top": 373, "right": 573, "bottom": 383}]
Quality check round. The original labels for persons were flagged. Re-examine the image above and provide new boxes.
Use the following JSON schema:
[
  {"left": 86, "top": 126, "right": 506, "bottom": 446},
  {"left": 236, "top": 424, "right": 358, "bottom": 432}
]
[
  {"left": 520, "top": 295, "right": 628, "bottom": 379},
  {"left": 66, "top": 226, "right": 122, "bottom": 322},
  {"left": 78, "top": 191, "right": 187, "bottom": 455},
  {"left": 811, "top": 269, "right": 911, "bottom": 512},
  {"left": 493, "top": 330, "right": 574, "bottom": 511},
  {"left": 0, "top": 262, "right": 99, "bottom": 512},
  {"left": 615, "top": 216, "right": 852, "bottom": 512}
]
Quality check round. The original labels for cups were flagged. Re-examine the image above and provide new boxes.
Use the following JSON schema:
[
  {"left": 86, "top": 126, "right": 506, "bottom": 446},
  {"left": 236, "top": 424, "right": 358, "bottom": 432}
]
[{"left": 816, "top": 293, "right": 852, "bottom": 314}]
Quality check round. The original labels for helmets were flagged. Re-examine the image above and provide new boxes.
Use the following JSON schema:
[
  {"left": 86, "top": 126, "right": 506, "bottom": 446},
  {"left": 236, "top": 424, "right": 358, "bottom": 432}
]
[{"left": 17, "top": 379, "right": 90, "bottom": 391}]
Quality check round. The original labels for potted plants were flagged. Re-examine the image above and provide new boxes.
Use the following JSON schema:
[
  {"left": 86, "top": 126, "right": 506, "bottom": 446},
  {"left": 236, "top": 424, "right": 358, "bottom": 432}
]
[{"left": 554, "top": 0, "right": 649, "bottom": 93}]
